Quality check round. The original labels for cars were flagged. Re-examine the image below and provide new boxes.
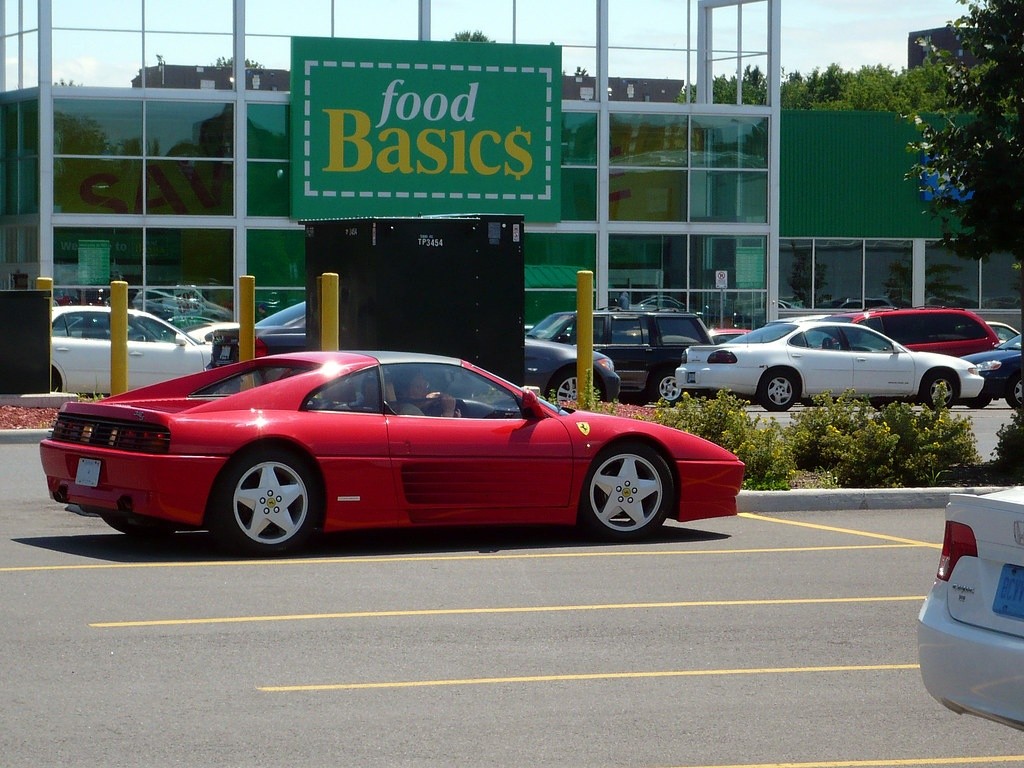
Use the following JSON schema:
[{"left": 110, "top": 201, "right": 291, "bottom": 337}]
[
  {"left": 51, "top": 296, "right": 306, "bottom": 394},
  {"left": 633, "top": 295, "right": 696, "bottom": 314},
  {"left": 525, "top": 307, "right": 1024, "bottom": 412},
  {"left": 778, "top": 295, "right": 1022, "bottom": 308},
  {"left": 131, "top": 285, "right": 234, "bottom": 323},
  {"left": 211, "top": 299, "right": 620, "bottom": 404}
]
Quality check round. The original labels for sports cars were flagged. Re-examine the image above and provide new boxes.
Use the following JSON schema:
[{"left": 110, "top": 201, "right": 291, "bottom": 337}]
[{"left": 38, "top": 350, "right": 747, "bottom": 561}]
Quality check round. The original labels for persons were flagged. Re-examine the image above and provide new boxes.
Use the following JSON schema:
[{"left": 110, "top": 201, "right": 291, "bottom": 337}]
[
  {"left": 258, "top": 303, "right": 268, "bottom": 318},
  {"left": 390, "top": 367, "right": 461, "bottom": 418}
]
